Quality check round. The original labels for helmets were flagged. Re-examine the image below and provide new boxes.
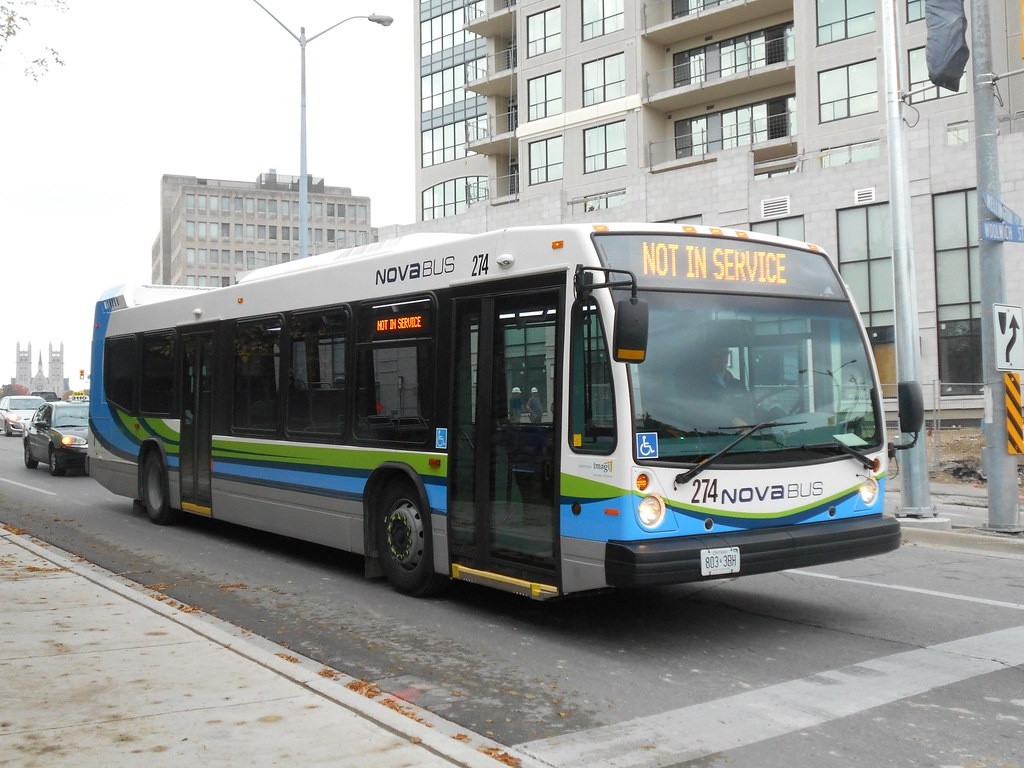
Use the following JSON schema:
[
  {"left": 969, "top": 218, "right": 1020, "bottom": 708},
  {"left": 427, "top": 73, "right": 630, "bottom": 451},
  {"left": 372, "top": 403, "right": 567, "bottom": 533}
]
[
  {"left": 512, "top": 387, "right": 521, "bottom": 393},
  {"left": 531, "top": 387, "right": 538, "bottom": 393}
]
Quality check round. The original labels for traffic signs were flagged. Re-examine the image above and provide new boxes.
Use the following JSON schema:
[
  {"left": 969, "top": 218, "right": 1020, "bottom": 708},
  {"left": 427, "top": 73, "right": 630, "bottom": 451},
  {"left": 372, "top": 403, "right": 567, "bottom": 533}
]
[{"left": 980, "top": 191, "right": 1024, "bottom": 242}]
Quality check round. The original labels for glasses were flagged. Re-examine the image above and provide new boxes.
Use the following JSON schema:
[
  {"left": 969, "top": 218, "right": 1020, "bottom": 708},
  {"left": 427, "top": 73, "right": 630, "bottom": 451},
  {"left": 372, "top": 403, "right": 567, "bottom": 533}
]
[{"left": 714, "top": 352, "right": 728, "bottom": 357}]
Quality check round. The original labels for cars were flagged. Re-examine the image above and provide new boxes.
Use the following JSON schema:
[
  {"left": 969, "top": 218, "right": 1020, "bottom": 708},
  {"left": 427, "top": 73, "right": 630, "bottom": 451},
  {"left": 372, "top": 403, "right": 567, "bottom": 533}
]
[{"left": 0, "top": 392, "right": 90, "bottom": 476}]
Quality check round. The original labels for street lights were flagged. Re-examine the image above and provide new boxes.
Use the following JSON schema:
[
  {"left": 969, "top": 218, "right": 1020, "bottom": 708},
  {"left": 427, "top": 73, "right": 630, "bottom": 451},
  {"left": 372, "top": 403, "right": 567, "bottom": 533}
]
[{"left": 251, "top": 0, "right": 394, "bottom": 260}]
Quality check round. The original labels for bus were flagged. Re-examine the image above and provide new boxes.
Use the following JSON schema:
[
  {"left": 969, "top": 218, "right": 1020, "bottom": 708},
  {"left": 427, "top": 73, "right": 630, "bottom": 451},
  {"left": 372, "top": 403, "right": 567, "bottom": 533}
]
[{"left": 84, "top": 221, "right": 903, "bottom": 603}]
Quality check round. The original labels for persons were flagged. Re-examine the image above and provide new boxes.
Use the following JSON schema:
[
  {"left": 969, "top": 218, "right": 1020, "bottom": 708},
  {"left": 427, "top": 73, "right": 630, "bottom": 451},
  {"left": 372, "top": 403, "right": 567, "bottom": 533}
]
[
  {"left": 525, "top": 387, "right": 543, "bottom": 423},
  {"left": 509, "top": 386, "right": 522, "bottom": 423},
  {"left": 707, "top": 342, "right": 754, "bottom": 437}
]
